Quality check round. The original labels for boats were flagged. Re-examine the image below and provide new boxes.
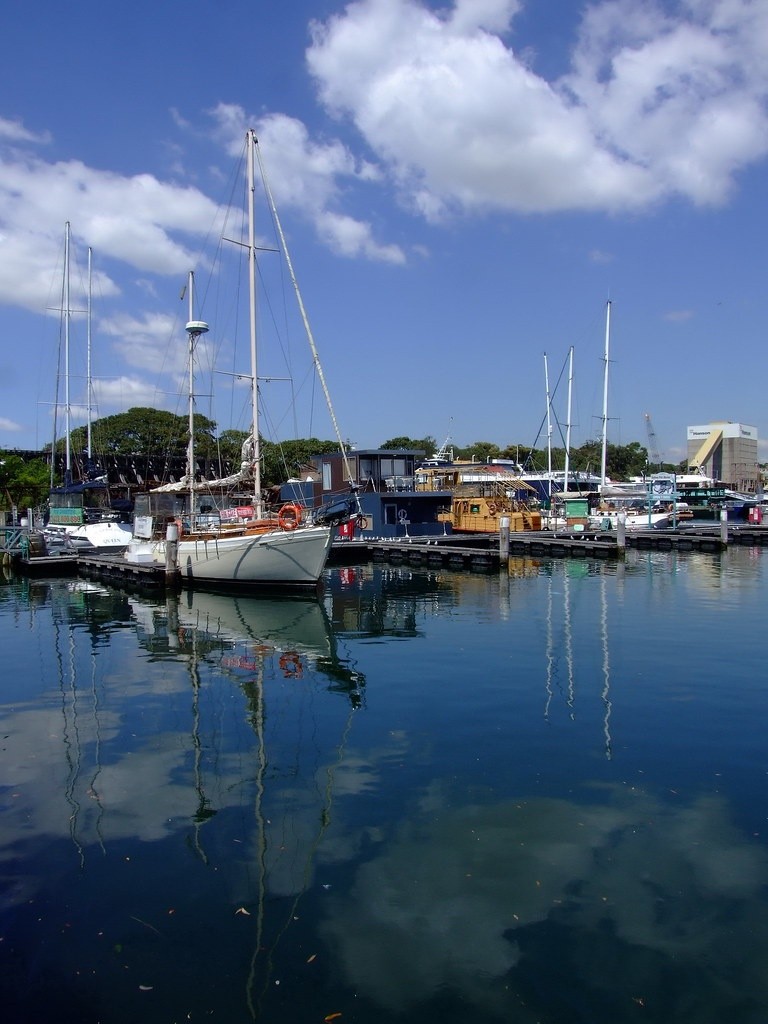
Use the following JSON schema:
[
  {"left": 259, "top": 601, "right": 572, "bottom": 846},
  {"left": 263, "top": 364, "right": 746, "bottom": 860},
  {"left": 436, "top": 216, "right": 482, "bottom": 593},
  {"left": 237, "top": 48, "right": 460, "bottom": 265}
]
[
  {"left": 415, "top": 414, "right": 602, "bottom": 542},
  {"left": 281, "top": 449, "right": 490, "bottom": 548}
]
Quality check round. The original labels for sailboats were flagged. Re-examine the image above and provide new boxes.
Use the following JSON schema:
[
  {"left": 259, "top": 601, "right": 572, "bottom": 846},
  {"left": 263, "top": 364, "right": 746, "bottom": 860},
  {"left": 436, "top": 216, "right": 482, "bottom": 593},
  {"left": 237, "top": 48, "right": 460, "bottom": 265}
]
[
  {"left": 518, "top": 297, "right": 768, "bottom": 536},
  {"left": 172, "top": 590, "right": 370, "bottom": 1019},
  {"left": 31, "top": 128, "right": 366, "bottom": 585}
]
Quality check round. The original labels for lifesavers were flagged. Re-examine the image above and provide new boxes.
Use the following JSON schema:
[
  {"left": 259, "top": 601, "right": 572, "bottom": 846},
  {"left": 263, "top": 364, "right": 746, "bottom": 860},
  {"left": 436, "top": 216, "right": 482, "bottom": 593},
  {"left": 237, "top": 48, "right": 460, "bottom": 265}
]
[
  {"left": 278, "top": 505, "right": 301, "bottom": 530},
  {"left": 176, "top": 519, "right": 183, "bottom": 537},
  {"left": 357, "top": 518, "right": 368, "bottom": 529},
  {"left": 280, "top": 652, "right": 302, "bottom": 679}
]
